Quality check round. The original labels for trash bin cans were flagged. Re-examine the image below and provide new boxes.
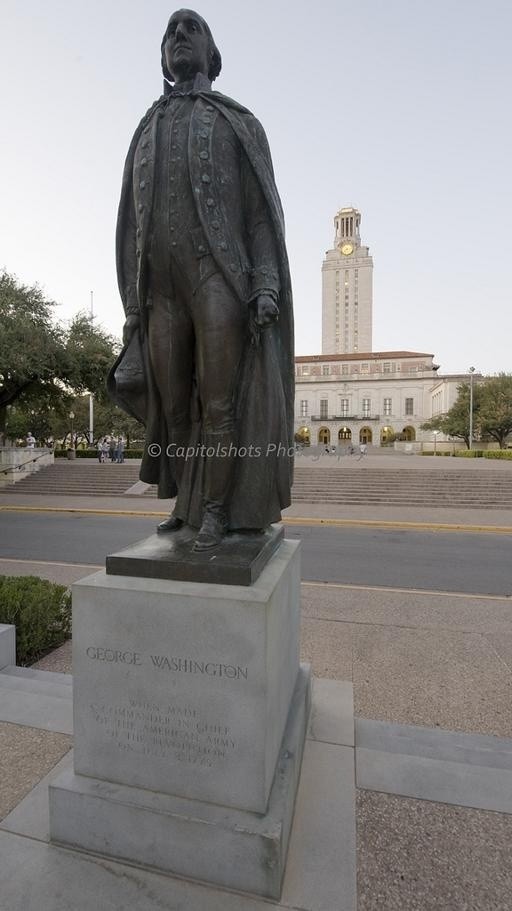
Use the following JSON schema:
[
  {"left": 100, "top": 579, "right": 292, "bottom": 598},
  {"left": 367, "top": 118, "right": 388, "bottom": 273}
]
[{"left": 67, "top": 448, "right": 75, "bottom": 460}]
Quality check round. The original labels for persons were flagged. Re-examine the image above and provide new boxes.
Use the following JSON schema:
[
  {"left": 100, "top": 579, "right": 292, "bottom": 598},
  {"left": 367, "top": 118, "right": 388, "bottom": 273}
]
[
  {"left": 105, "top": 8, "right": 299, "bottom": 555},
  {"left": 15, "top": 430, "right": 134, "bottom": 465}
]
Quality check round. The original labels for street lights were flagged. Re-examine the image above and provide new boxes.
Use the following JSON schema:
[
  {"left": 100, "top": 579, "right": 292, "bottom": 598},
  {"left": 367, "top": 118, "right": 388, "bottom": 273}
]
[
  {"left": 433, "top": 429, "right": 441, "bottom": 456},
  {"left": 468, "top": 365, "right": 476, "bottom": 450},
  {"left": 67, "top": 409, "right": 76, "bottom": 459}
]
